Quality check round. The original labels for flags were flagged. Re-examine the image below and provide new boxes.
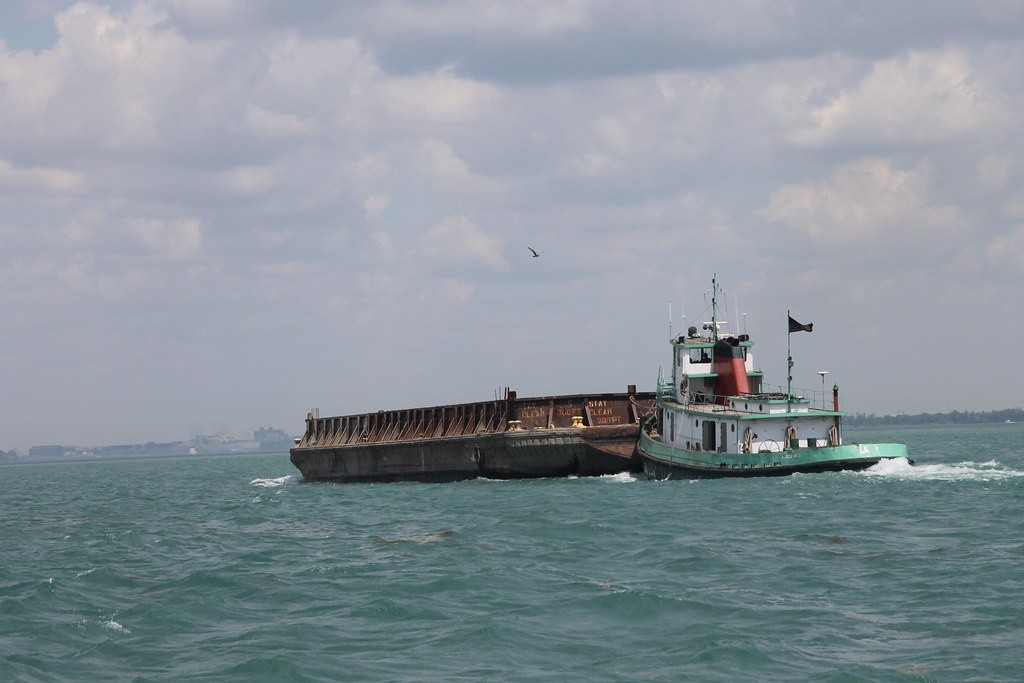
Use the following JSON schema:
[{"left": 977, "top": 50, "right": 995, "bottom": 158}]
[{"left": 789, "top": 313, "right": 813, "bottom": 332}]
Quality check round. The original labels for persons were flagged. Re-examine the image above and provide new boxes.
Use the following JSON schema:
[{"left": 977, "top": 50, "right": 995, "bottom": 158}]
[{"left": 701, "top": 353, "right": 711, "bottom": 363}]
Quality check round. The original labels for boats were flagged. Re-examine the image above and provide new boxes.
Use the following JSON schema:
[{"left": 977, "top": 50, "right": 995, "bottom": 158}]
[
  {"left": 637, "top": 273, "right": 916, "bottom": 480},
  {"left": 290, "top": 384, "right": 656, "bottom": 483}
]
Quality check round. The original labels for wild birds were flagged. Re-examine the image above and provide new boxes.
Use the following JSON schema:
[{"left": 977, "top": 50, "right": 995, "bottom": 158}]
[{"left": 528, "top": 246, "right": 547, "bottom": 258}]
